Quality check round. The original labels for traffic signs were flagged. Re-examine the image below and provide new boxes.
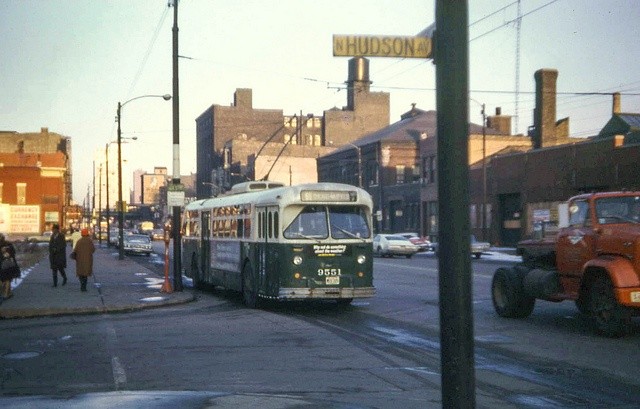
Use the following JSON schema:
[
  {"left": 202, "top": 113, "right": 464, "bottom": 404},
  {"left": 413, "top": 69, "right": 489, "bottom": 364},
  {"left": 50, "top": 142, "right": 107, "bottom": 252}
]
[{"left": 332, "top": 33, "right": 433, "bottom": 58}]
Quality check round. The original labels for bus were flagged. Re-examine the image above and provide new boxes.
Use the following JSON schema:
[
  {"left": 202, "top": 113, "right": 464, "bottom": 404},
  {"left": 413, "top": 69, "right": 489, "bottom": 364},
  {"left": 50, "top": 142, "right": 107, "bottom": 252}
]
[
  {"left": 178, "top": 181, "right": 377, "bottom": 310},
  {"left": 137, "top": 221, "right": 153, "bottom": 234}
]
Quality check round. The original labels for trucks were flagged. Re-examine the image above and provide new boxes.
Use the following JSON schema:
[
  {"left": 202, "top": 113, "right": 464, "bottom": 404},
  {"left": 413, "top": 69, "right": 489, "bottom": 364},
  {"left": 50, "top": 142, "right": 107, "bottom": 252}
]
[{"left": 490, "top": 187, "right": 640, "bottom": 339}]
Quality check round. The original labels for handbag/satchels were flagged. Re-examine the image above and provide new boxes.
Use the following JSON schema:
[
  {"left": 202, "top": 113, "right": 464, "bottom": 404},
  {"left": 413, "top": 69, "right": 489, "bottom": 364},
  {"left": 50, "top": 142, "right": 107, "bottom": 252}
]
[{"left": 70, "top": 252, "right": 77, "bottom": 260}]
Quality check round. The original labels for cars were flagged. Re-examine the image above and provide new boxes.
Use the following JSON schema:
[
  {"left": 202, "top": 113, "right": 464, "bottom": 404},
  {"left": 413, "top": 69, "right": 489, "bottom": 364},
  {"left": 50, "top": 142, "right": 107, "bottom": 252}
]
[
  {"left": 109, "top": 231, "right": 124, "bottom": 244},
  {"left": 124, "top": 234, "right": 153, "bottom": 256},
  {"left": 150, "top": 229, "right": 166, "bottom": 241},
  {"left": 405, "top": 233, "right": 440, "bottom": 252},
  {"left": 94, "top": 230, "right": 108, "bottom": 240},
  {"left": 116, "top": 233, "right": 126, "bottom": 248},
  {"left": 372, "top": 233, "right": 417, "bottom": 259}
]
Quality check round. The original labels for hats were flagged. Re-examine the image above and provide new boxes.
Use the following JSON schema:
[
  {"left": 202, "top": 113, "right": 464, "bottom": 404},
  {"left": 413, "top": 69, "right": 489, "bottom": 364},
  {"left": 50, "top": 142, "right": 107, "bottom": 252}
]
[{"left": 81, "top": 229, "right": 88, "bottom": 236}]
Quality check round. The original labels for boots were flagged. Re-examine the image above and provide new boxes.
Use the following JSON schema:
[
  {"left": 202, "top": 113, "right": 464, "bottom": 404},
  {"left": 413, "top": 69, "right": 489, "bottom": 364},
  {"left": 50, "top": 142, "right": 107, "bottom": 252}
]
[
  {"left": 53, "top": 276, "right": 57, "bottom": 287},
  {"left": 62, "top": 272, "right": 67, "bottom": 286},
  {"left": 81, "top": 280, "right": 87, "bottom": 291}
]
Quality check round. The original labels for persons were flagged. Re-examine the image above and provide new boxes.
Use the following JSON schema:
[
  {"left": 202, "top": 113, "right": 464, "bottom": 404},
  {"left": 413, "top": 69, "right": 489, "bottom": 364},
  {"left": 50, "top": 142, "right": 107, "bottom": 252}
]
[
  {"left": 48, "top": 224, "right": 67, "bottom": 287},
  {"left": 71, "top": 228, "right": 82, "bottom": 249},
  {"left": 625, "top": 200, "right": 640, "bottom": 221},
  {"left": 74, "top": 227, "right": 95, "bottom": 292},
  {"left": 0, "top": 232, "right": 21, "bottom": 300}
]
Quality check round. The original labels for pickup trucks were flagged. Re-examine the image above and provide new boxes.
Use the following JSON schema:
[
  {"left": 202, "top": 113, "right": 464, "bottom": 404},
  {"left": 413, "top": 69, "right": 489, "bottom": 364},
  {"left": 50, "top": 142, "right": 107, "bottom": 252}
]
[{"left": 470, "top": 234, "right": 490, "bottom": 258}]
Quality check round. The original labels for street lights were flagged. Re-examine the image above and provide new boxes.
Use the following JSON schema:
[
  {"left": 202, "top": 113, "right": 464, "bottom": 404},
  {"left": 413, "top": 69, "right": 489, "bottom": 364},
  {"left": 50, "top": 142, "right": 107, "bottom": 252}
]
[
  {"left": 98, "top": 157, "right": 128, "bottom": 245},
  {"left": 230, "top": 172, "right": 251, "bottom": 181},
  {"left": 328, "top": 141, "right": 363, "bottom": 187},
  {"left": 202, "top": 182, "right": 224, "bottom": 191},
  {"left": 105, "top": 137, "right": 137, "bottom": 248},
  {"left": 87, "top": 182, "right": 106, "bottom": 236},
  {"left": 115, "top": 93, "right": 172, "bottom": 259}
]
